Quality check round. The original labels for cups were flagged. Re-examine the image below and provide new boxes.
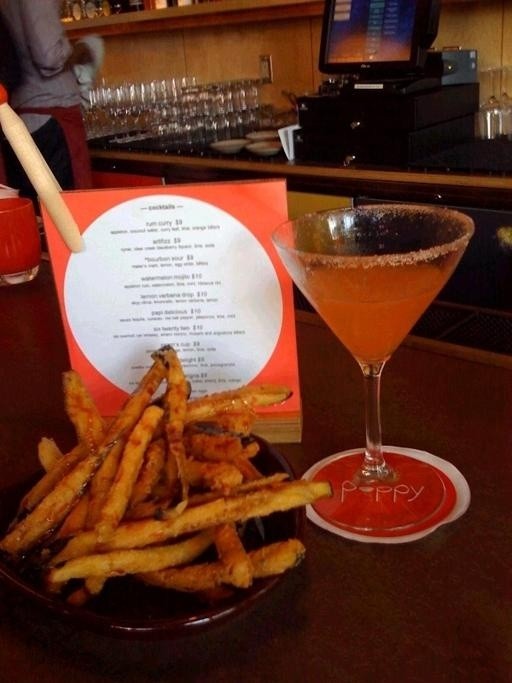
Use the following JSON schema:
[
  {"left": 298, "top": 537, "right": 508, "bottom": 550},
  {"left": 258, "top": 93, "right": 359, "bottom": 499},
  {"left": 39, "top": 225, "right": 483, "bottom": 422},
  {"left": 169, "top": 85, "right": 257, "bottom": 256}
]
[{"left": 0, "top": 197, "right": 41, "bottom": 285}]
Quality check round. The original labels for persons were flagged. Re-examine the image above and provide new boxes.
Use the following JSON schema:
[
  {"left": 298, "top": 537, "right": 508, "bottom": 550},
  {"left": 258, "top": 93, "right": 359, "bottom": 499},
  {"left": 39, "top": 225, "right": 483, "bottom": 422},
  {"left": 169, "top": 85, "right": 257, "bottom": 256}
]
[{"left": 1, "top": 0, "right": 93, "bottom": 198}]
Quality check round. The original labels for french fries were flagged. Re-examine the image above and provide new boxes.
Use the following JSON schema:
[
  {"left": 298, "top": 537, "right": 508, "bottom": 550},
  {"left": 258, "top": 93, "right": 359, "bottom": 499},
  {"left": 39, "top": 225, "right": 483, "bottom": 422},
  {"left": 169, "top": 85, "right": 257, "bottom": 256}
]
[{"left": 1, "top": 344, "right": 334, "bottom": 607}]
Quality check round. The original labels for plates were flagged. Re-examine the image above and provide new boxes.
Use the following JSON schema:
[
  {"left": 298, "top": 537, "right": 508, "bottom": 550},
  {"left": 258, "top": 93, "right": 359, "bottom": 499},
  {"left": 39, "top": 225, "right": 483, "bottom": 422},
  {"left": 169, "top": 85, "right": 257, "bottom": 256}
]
[
  {"left": 211, "top": 130, "right": 283, "bottom": 157},
  {"left": 1, "top": 432, "right": 301, "bottom": 641}
]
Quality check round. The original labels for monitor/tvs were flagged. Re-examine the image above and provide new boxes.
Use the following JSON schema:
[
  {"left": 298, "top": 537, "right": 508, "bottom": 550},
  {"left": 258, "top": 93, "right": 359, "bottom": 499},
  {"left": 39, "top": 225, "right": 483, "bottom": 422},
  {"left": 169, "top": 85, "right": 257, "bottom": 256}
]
[{"left": 319, "top": 0, "right": 439, "bottom": 94}]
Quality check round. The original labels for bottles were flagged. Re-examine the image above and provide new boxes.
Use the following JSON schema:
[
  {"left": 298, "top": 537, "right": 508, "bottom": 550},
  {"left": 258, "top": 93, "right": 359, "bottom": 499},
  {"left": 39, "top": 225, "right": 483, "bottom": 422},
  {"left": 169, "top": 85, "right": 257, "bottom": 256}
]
[{"left": 65, "top": 1, "right": 142, "bottom": 21}]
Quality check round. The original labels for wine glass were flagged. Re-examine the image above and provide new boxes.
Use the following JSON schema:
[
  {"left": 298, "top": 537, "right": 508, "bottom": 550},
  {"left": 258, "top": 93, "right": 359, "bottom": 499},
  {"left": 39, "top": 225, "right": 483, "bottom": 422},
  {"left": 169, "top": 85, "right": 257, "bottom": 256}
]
[
  {"left": 272, "top": 206, "right": 477, "bottom": 532},
  {"left": 82, "top": 77, "right": 262, "bottom": 146}
]
